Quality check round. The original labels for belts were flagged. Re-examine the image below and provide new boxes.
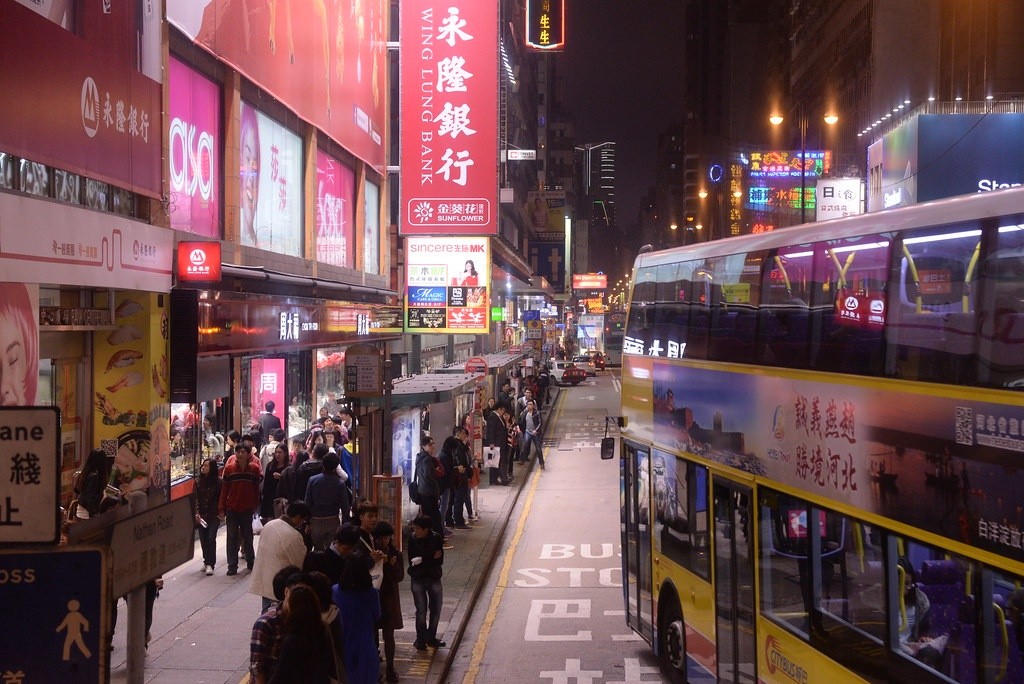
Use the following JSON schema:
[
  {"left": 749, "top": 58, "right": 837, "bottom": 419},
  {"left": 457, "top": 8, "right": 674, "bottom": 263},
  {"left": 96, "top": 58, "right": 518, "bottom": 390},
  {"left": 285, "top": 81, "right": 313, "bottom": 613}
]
[{"left": 312, "top": 516, "right": 337, "bottom": 519}]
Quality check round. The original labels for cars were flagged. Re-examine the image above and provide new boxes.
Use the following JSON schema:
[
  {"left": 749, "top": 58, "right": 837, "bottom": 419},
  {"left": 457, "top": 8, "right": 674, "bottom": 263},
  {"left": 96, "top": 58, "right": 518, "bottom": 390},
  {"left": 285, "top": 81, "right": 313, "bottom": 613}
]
[
  {"left": 584, "top": 351, "right": 606, "bottom": 370},
  {"left": 546, "top": 359, "right": 586, "bottom": 386},
  {"left": 572, "top": 356, "right": 596, "bottom": 376}
]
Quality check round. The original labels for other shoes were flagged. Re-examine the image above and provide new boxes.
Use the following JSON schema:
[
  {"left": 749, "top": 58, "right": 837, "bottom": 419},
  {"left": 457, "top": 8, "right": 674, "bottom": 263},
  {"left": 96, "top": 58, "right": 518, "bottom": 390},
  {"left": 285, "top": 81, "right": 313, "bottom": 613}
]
[
  {"left": 426, "top": 638, "right": 446, "bottom": 646},
  {"left": 442, "top": 536, "right": 448, "bottom": 540},
  {"left": 413, "top": 640, "right": 427, "bottom": 651},
  {"left": 205, "top": 565, "right": 214, "bottom": 575},
  {"left": 501, "top": 476, "right": 514, "bottom": 486},
  {"left": 442, "top": 542, "right": 454, "bottom": 549},
  {"left": 455, "top": 526, "right": 473, "bottom": 531},
  {"left": 200, "top": 561, "right": 207, "bottom": 571},
  {"left": 385, "top": 667, "right": 399, "bottom": 684},
  {"left": 227, "top": 569, "right": 237, "bottom": 575}
]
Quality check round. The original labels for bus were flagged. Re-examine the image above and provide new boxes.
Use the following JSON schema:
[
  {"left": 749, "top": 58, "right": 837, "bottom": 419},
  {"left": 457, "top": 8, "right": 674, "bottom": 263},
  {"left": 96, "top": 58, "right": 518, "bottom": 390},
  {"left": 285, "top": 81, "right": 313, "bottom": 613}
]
[
  {"left": 601, "top": 188, "right": 1024, "bottom": 684},
  {"left": 578, "top": 312, "right": 626, "bottom": 366}
]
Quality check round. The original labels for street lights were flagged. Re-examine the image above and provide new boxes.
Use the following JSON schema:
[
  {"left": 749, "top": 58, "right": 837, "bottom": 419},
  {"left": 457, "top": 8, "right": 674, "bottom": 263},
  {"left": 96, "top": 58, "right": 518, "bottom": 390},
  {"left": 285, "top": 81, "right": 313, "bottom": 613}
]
[
  {"left": 669, "top": 221, "right": 703, "bottom": 246},
  {"left": 768, "top": 111, "right": 838, "bottom": 223},
  {"left": 700, "top": 188, "right": 743, "bottom": 238}
]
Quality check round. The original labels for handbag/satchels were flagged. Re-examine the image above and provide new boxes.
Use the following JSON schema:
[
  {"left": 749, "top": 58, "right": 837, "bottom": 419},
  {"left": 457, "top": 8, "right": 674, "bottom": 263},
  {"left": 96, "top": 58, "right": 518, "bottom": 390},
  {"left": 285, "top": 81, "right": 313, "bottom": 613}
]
[
  {"left": 482, "top": 446, "right": 501, "bottom": 468},
  {"left": 467, "top": 462, "right": 480, "bottom": 488},
  {"left": 251, "top": 513, "right": 264, "bottom": 535},
  {"left": 408, "top": 467, "right": 422, "bottom": 505}
]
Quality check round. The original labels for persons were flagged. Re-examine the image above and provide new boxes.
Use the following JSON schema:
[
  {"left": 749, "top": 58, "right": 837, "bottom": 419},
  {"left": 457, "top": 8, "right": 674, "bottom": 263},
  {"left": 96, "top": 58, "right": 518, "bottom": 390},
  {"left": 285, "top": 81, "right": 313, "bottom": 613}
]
[
  {"left": 217, "top": 443, "right": 260, "bottom": 576},
  {"left": 850, "top": 557, "right": 932, "bottom": 665},
  {"left": 408, "top": 514, "right": 446, "bottom": 649},
  {"left": 171, "top": 354, "right": 549, "bottom": 684},
  {"left": 66, "top": 501, "right": 164, "bottom": 657},
  {"left": 192, "top": 458, "right": 222, "bottom": 575},
  {"left": 461, "top": 260, "right": 478, "bottom": 285},
  {"left": 0, "top": 282, "right": 38, "bottom": 406}
]
